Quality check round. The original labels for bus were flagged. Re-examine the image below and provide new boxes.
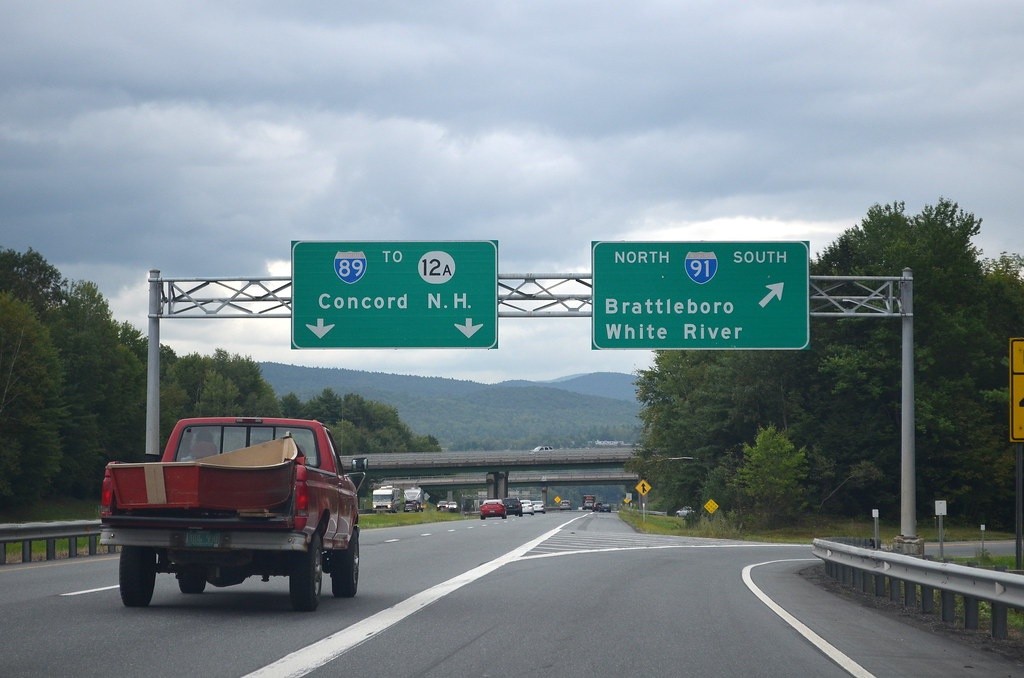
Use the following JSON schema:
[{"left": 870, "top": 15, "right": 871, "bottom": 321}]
[{"left": 373, "top": 486, "right": 401, "bottom": 514}]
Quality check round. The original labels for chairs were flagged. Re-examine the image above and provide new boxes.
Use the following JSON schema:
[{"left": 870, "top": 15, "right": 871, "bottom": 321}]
[{"left": 192, "top": 442, "right": 214, "bottom": 459}]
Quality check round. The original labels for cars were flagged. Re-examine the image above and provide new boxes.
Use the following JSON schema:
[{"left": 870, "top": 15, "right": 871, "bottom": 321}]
[
  {"left": 596, "top": 502, "right": 612, "bottom": 513},
  {"left": 480, "top": 499, "right": 509, "bottom": 520},
  {"left": 560, "top": 499, "right": 572, "bottom": 510},
  {"left": 503, "top": 497, "right": 524, "bottom": 517},
  {"left": 436, "top": 501, "right": 449, "bottom": 512},
  {"left": 530, "top": 446, "right": 555, "bottom": 454},
  {"left": 520, "top": 499, "right": 535, "bottom": 515},
  {"left": 532, "top": 501, "right": 547, "bottom": 514},
  {"left": 447, "top": 501, "right": 461, "bottom": 513}
]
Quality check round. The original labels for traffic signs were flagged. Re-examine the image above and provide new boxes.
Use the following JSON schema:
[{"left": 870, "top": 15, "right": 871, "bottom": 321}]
[
  {"left": 289, "top": 239, "right": 501, "bottom": 352},
  {"left": 590, "top": 240, "right": 816, "bottom": 351}
]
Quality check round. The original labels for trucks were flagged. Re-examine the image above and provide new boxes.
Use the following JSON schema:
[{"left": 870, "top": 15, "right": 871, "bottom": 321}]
[
  {"left": 583, "top": 495, "right": 596, "bottom": 510},
  {"left": 403, "top": 488, "right": 425, "bottom": 512}
]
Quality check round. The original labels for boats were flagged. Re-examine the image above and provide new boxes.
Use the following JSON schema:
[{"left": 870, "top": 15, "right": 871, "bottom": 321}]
[{"left": 105, "top": 430, "right": 301, "bottom": 511}]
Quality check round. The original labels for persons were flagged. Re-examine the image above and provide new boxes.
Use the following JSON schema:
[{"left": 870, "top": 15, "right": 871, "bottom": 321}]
[{"left": 196, "top": 430, "right": 212, "bottom": 443}]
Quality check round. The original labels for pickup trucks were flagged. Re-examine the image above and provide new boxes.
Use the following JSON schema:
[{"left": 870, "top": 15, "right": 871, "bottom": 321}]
[{"left": 99, "top": 416, "right": 368, "bottom": 610}]
